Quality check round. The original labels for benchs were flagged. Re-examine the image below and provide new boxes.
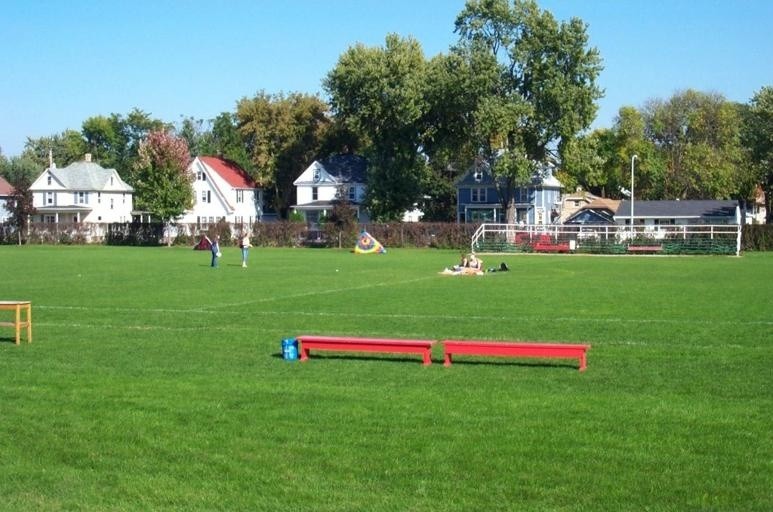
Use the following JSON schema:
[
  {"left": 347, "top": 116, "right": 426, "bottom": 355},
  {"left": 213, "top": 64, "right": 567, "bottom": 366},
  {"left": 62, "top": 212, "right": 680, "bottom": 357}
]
[
  {"left": 296, "top": 335, "right": 437, "bottom": 365},
  {"left": 439, "top": 340, "right": 590, "bottom": 372},
  {"left": 533, "top": 244, "right": 568, "bottom": 253},
  {"left": 626, "top": 245, "right": 661, "bottom": 255}
]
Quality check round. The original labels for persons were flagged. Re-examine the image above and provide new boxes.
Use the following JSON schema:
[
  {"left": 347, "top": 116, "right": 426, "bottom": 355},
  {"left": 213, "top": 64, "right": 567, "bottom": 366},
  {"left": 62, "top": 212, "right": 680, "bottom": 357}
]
[
  {"left": 241, "top": 232, "right": 253, "bottom": 267},
  {"left": 211, "top": 234, "right": 221, "bottom": 267},
  {"left": 453, "top": 253, "right": 483, "bottom": 272}
]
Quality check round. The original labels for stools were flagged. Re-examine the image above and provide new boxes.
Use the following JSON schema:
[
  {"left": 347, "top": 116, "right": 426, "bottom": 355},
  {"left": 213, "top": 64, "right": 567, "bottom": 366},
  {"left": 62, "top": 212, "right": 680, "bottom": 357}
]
[{"left": 0, "top": 301, "right": 32, "bottom": 346}]
